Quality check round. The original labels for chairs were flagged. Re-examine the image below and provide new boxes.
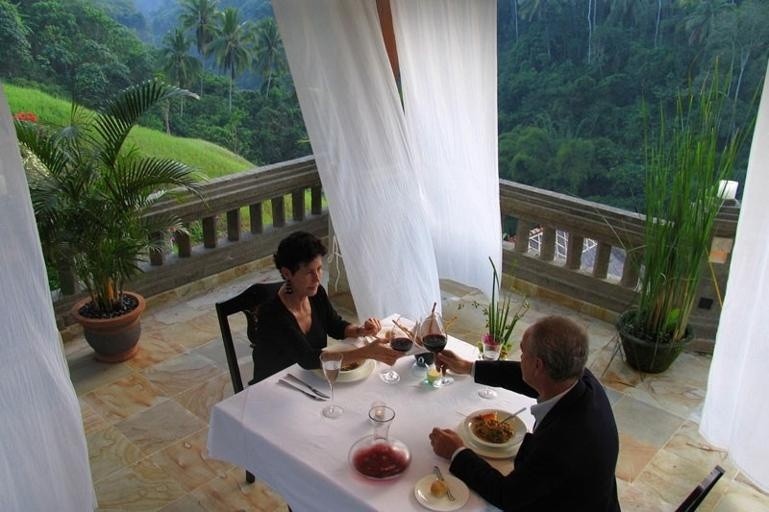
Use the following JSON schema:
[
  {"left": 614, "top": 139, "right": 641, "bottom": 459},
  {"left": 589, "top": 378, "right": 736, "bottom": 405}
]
[
  {"left": 214, "top": 281, "right": 284, "bottom": 483},
  {"left": 674, "top": 464, "right": 725, "bottom": 511}
]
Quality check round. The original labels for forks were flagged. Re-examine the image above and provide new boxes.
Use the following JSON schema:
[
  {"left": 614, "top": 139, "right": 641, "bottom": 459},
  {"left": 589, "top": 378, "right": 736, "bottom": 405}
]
[
  {"left": 280, "top": 380, "right": 326, "bottom": 401},
  {"left": 498, "top": 408, "right": 527, "bottom": 422}
]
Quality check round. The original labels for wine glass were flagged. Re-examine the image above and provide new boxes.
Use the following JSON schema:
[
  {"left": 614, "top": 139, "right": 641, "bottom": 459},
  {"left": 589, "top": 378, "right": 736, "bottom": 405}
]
[
  {"left": 477, "top": 335, "right": 505, "bottom": 400},
  {"left": 379, "top": 314, "right": 419, "bottom": 384},
  {"left": 420, "top": 312, "right": 454, "bottom": 388},
  {"left": 320, "top": 352, "right": 343, "bottom": 418}
]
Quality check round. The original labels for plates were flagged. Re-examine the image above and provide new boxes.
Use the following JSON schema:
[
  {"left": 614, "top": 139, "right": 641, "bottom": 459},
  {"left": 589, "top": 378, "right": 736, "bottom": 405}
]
[
  {"left": 457, "top": 418, "right": 529, "bottom": 457},
  {"left": 415, "top": 473, "right": 469, "bottom": 511},
  {"left": 313, "top": 358, "right": 377, "bottom": 383}
]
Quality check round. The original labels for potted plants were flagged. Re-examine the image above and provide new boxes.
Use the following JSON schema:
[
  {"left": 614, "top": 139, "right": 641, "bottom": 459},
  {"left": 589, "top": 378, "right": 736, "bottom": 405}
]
[
  {"left": 614, "top": 39, "right": 764, "bottom": 373},
  {"left": 11, "top": 78, "right": 211, "bottom": 364}
]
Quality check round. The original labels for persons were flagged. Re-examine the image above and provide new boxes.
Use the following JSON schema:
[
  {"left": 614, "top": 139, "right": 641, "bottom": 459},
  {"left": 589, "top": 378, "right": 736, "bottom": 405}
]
[
  {"left": 430, "top": 315, "right": 621, "bottom": 512},
  {"left": 253, "top": 230, "right": 405, "bottom": 386}
]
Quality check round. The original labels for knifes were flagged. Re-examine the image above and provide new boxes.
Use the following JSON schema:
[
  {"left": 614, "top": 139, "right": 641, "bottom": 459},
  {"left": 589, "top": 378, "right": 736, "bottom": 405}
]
[
  {"left": 434, "top": 465, "right": 456, "bottom": 501},
  {"left": 287, "top": 374, "right": 330, "bottom": 398}
]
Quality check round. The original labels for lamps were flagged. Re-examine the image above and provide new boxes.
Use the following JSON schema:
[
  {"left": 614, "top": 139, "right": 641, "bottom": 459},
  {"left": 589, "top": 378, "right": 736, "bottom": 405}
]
[{"left": 716, "top": 179, "right": 739, "bottom": 199}]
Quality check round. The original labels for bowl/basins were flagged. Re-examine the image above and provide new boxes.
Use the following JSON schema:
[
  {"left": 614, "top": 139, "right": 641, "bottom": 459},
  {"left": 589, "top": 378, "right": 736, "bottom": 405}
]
[
  {"left": 466, "top": 409, "right": 526, "bottom": 447},
  {"left": 320, "top": 344, "right": 367, "bottom": 373}
]
